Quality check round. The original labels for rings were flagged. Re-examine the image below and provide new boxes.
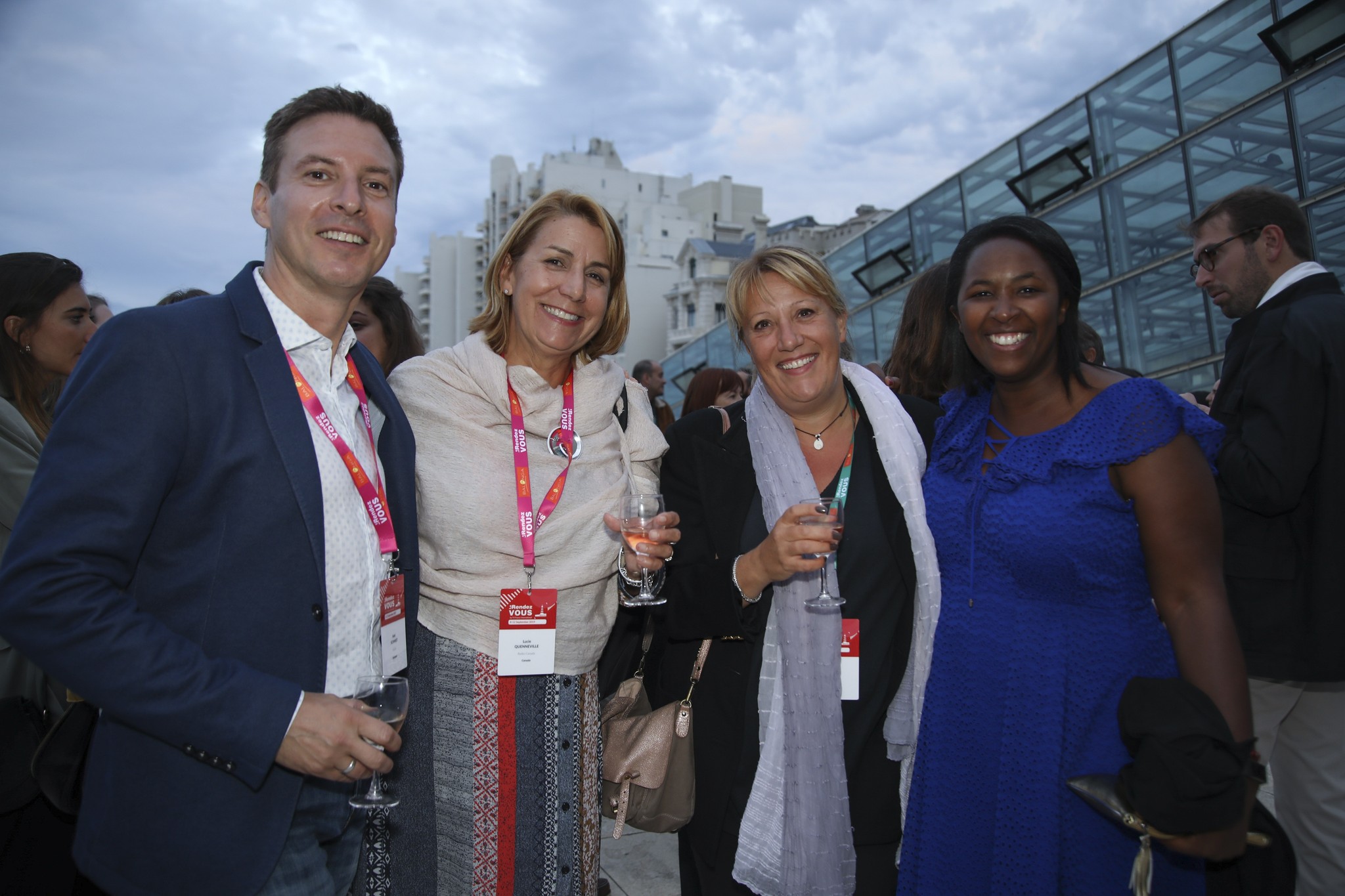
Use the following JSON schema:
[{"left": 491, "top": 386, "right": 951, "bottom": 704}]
[
  {"left": 664, "top": 549, "right": 674, "bottom": 561},
  {"left": 341, "top": 757, "right": 356, "bottom": 774}
]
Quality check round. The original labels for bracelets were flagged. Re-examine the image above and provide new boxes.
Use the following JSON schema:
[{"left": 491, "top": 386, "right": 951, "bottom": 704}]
[
  {"left": 732, "top": 554, "right": 762, "bottom": 603},
  {"left": 618, "top": 544, "right": 656, "bottom": 587},
  {"left": 1241, "top": 736, "right": 1261, "bottom": 763}
]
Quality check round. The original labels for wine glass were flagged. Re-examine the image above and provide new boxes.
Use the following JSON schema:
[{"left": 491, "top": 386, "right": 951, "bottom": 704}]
[
  {"left": 799, "top": 495, "right": 846, "bottom": 609},
  {"left": 348, "top": 676, "right": 408, "bottom": 811},
  {"left": 619, "top": 494, "right": 668, "bottom": 608}
]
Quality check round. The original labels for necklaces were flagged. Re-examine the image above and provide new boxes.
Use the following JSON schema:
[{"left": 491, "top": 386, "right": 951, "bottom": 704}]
[{"left": 795, "top": 393, "right": 849, "bottom": 450}]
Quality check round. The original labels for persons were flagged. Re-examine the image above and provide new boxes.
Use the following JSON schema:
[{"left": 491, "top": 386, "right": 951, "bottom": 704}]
[
  {"left": 866, "top": 215, "right": 1270, "bottom": 896},
  {"left": 0, "top": 83, "right": 423, "bottom": 896},
  {"left": 349, "top": 276, "right": 423, "bottom": 379},
  {"left": 640, "top": 245, "right": 948, "bottom": 896},
  {"left": 87, "top": 293, "right": 114, "bottom": 329},
  {"left": 633, "top": 356, "right": 1223, "bottom": 421},
  {"left": 1183, "top": 184, "right": 1345, "bottom": 896},
  {"left": 0, "top": 251, "right": 98, "bottom": 895},
  {"left": 367, "top": 189, "right": 681, "bottom": 896},
  {"left": 885, "top": 263, "right": 949, "bottom": 412}
]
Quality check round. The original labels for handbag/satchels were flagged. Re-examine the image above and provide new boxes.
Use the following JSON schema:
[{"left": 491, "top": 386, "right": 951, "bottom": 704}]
[
  {"left": 599, "top": 677, "right": 696, "bottom": 840},
  {"left": 1066, "top": 773, "right": 1272, "bottom": 896},
  {"left": 597, "top": 603, "right": 647, "bottom": 699}
]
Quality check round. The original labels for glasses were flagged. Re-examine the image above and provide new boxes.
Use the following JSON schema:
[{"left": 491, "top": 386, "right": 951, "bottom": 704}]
[{"left": 1190, "top": 226, "right": 1268, "bottom": 279}]
